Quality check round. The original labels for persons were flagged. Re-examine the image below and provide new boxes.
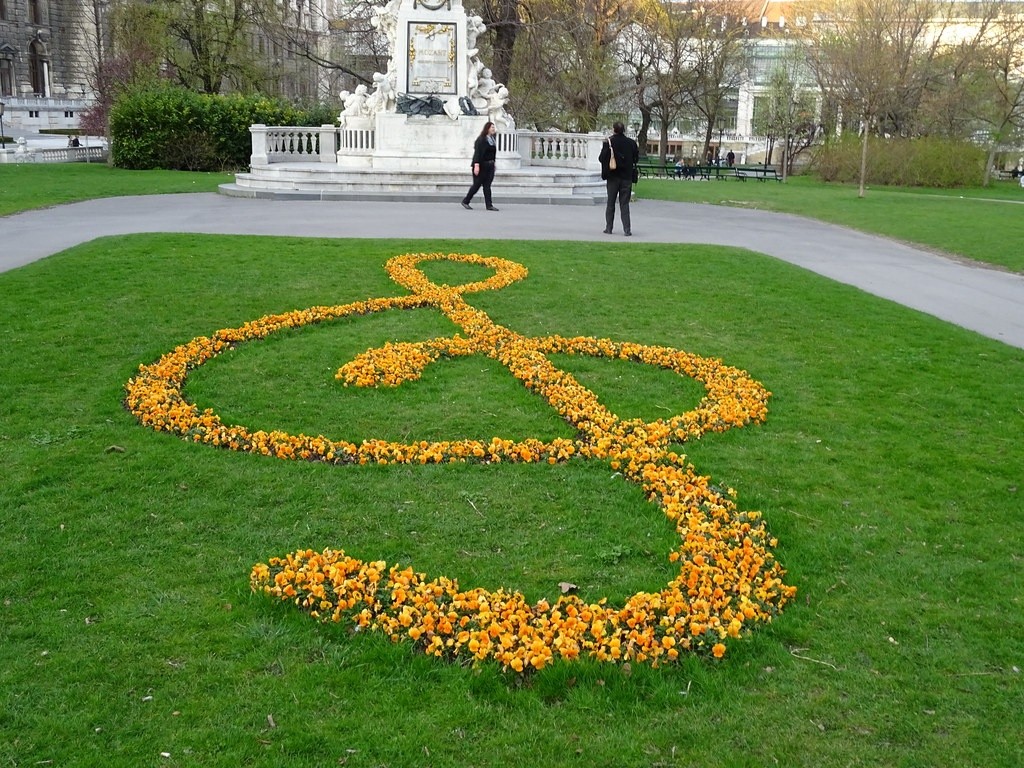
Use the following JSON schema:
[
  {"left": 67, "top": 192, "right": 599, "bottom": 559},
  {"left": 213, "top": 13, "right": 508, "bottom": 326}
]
[
  {"left": 1011, "top": 167, "right": 1024, "bottom": 180},
  {"left": 461, "top": 122, "right": 499, "bottom": 211},
  {"left": 339, "top": 67, "right": 395, "bottom": 128},
  {"left": 675, "top": 158, "right": 696, "bottom": 181},
  {"left": 467, "top": 16, "right": 510, "bottom": 128},
  {"left": 599, "top": 121, "right": 640, "bottom": 236},
  {"left": 727, "top": 149, "right": 735, "bottom": 167}
]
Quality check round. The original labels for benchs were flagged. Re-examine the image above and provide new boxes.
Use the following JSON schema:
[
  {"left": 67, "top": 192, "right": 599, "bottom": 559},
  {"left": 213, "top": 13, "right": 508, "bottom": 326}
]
[{"left": 632, "top": 165, "right": 776, "bottom": 182}]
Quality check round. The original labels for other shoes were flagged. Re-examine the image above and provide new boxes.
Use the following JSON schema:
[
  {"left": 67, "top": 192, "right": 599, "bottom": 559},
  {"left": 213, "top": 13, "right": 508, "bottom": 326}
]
[
  {"left": 624, "top": 230, "right": 632, "bottom": 236},
  {"left": 461, "top": 201, "right": 472, "bottom": 209},
  {"left": 487, "top": 207, "right": 499, "bottom": 211},
  {"left": 604, "top": 230, "right": 612, "bottom": 234}
]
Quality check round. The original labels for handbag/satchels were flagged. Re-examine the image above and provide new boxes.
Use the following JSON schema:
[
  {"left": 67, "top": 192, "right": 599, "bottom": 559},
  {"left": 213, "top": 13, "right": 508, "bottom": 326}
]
[
  {"left": 632, "top": 169, "right": 638, "bottom": 183},
  {"left": 608, "top": 138, "right": 616, "bottom": 169}
]
